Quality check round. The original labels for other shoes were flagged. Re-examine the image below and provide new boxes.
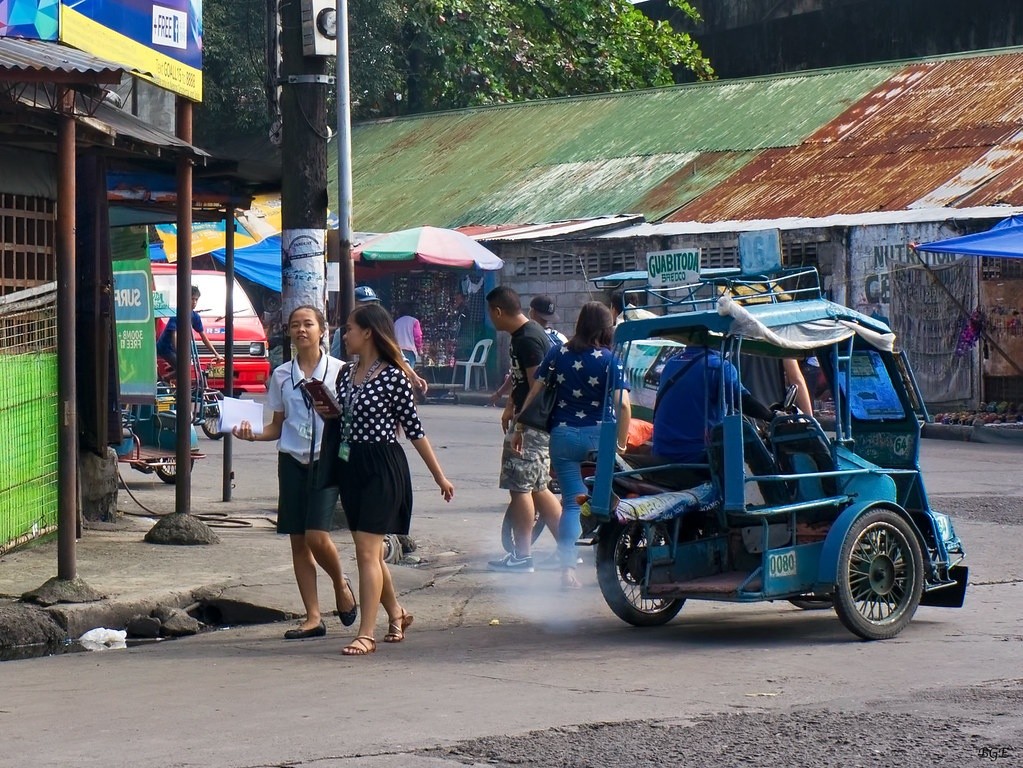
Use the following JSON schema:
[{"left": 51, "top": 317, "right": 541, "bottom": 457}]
[
  {"left": 192, "top": 416, "right": 206, "bottom": 424},
  {"left": 285, "top": 621, "right": 326, "bottom": 639},
  {"left": 336, "top": 577, "right": 358, "bottom": 627}
]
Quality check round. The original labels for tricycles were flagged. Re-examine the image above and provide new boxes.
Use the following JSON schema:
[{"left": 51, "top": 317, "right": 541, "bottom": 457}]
[
  {"left": 153, "top": 290, "right": 225, "bottom": 440},
  {"left": 108, "top": 387, "right": 206, "bottom": 483}
]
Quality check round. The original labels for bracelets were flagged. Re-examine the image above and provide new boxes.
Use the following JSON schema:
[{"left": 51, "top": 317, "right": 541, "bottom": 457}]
[
  {"left": 253, "top": 432, "right": 257, "bottom": 441},
  {"left": 505, "top": 400, "right": 513, "bottom": 404},
  {"left": 513, "top": 424, "right": 525, "bottom": 433},
  {"left": 496, "top": 390, "right": 502, "bottom": 398},
  {"left": 616, "top": 438, "right": 627, "bottom": 451}
]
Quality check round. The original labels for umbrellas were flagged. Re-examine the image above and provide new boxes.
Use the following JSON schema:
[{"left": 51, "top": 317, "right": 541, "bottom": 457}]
[
  {"left": 152, "top": 193, "right": 343, "bottom": 263},
  {"left": 348, "top": 226, "right": 506, "bottom": 297}
]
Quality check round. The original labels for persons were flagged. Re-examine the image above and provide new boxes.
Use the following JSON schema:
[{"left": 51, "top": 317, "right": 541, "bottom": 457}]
[
  {"left": 330, "top": 286, "right": 429, "bottom": 395},
  {"left": 311, "top": 305, "right": 454, "bottom": 657},
  {"left": 485, "top": 285, "right": 583, "bottom": 572},
  {"left": 233, "top": 306, "right": 359, "bottom": 640},
  {"left": 651, "top": 324, "right": 793, "bottom": 484},
  {"left": 156, "top": 286, "right": 225, "bottom": 384},
  {"left": 393, "top": 302, "right": 423, "bottom": 370},
  {"left": 731, "top": 351, "right": 815, "bottom": 417},
  {"left": 487, "top": 293, "right": 569, "bottom": 407},
  {"left": 510, "top": 302, "right": 632, "bottom": 568}
]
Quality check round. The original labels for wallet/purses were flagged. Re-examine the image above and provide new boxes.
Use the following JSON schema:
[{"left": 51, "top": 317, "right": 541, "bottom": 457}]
[{"left": 305, "top": 380, "right": 341, "bottom": 418}]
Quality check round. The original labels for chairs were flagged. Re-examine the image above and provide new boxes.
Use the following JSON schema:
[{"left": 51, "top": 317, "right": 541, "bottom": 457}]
[
  {"left": 711, "top": 410, "right": 858, "bottom": 521},
  {"left": 452, "top": 338, "right": 493, "bottom": 391}
]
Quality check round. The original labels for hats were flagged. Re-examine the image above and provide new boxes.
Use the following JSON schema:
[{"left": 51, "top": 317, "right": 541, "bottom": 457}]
[
  {"left": 354, "top": 285, "right": 381, "bottom": 302},
  {"left": 530, "top": 294, "right": 560, "bottom": 321}
]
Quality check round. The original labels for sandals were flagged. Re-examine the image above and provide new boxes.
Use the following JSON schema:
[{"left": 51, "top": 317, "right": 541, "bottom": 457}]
[
  {"left": 385, "top": 607, "right": 416, "bottom": 643},
  {"left": 560, "top": 574, "right": 582, "bottom": 592},
  {"left": 342, "top": 637, "right": 377, "bottom": 655}
]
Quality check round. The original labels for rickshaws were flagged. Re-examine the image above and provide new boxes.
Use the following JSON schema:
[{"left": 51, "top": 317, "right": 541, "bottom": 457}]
[{"left": 501, "top": 228, "right": 968, "bottom": 640}]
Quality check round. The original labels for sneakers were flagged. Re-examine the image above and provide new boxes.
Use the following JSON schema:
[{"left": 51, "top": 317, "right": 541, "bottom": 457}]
[
  {"left": 537, "top": 550, "right": 584, "bottom": 568},
  {"left": 488, "top": 552, "right": 535, "bottom": 572}
]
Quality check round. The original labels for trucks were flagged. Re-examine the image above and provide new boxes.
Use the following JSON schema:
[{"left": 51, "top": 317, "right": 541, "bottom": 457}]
[{"left": 151, "top": 263, "right": 271, "bottom": 400}]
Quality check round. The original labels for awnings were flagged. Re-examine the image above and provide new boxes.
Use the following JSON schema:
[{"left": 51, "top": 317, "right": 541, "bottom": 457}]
[
  {"left": 7, "top": 80, "right": 212, "bottom": 167},
  {"left": 0, "top": 36, "right": 153, "bottom": 83},
  {"left": 909, "top": 211, "right": 1023, "bottom": 377}
]
